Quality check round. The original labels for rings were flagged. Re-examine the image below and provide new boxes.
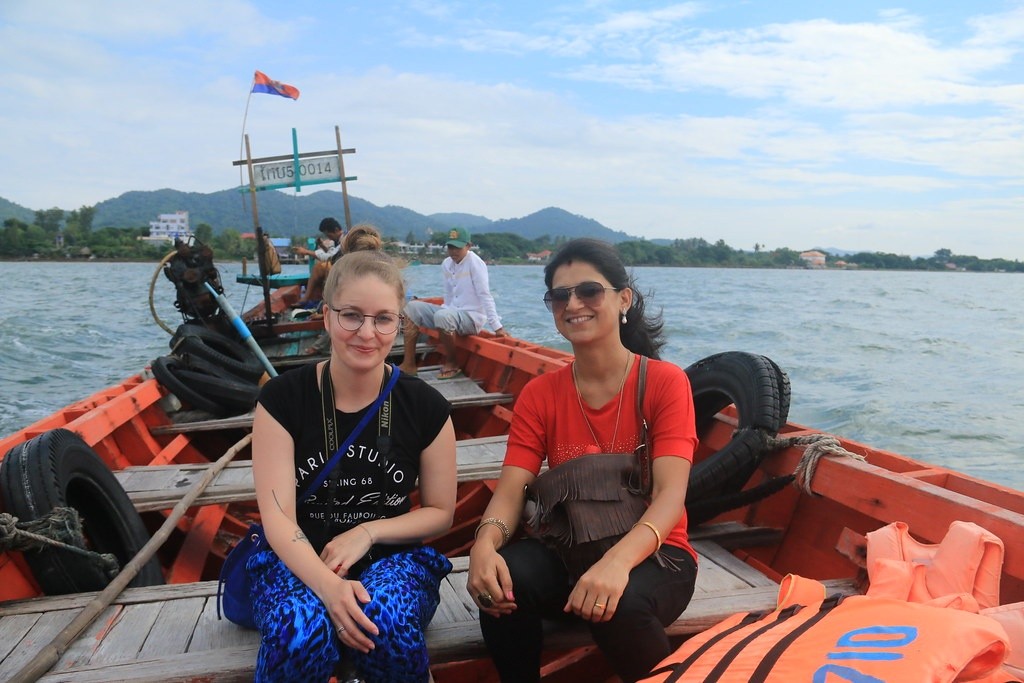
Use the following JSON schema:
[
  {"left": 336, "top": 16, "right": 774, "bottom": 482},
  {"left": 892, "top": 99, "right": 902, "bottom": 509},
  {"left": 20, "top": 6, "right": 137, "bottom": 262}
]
[
  {"left": 338, "top": 627, "right": 345, "bottom": 633},
  {"left": 595, "top": 604, "right": 606, "bottom": 609},
  {"left": 478, "top": 592, "right": 493, "bottom": 608}
]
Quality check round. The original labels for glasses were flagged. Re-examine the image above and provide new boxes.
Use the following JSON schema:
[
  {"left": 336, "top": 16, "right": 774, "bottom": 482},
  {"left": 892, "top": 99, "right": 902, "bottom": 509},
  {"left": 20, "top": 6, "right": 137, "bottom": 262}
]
[
  {"left": 331, "top": 306, "right": 405, "bottom": 335},
  {"left": 543, "top": 281, "right": 617, "bottom": 313}
]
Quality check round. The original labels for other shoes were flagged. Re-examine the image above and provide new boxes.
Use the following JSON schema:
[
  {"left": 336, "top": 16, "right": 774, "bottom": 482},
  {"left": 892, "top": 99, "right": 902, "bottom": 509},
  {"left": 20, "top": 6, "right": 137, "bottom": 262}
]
[
  {"left": 290, "top": 302, "right": 305, "bottom": 309},
  {"left": 306, "top": 347, "right": 322, "bottom": 355}
]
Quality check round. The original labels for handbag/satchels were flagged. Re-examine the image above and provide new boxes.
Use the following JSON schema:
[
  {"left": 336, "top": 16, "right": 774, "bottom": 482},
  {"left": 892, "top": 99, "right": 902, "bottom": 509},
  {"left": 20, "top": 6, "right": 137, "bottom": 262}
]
[
  {"left": 217, "top": 524, "right": 270, "bottom": 629},
  {"left": 524, "top": 355, "right": 685, "bottom": 575}
]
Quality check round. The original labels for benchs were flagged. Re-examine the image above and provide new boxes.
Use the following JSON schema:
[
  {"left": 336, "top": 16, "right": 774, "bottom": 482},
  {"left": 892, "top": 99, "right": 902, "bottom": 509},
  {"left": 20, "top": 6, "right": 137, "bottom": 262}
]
[
  {"left": 0, "top": 521, "right": 859, "bottom": 683},
  {"left": 153, "top": 362, "right": 516, "bottom": 434},
  {"left": 117, "top": 435, "right": 551, "bottom": 511}
]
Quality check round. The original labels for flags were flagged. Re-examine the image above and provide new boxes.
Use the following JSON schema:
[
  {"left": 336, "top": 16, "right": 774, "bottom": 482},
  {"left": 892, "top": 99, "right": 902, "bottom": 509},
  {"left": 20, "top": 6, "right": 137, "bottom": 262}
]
[{"left": 252, "top": 71, "right": 300, "bottom": 99}]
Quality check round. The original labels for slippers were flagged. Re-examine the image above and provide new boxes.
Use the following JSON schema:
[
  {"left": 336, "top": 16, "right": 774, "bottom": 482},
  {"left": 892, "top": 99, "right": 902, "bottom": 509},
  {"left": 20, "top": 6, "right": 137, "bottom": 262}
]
[{"left": 437, "top": 367, "right": 462, "bottom": 380}]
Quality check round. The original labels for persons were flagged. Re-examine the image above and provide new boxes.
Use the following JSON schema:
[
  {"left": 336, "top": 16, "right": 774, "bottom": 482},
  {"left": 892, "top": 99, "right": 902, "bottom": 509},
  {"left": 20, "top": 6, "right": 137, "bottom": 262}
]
[
  {"left": 466, "top": 238, "right": 699, "bottom": 683},
  {"left": 309, "top": 314, "right": 324, "bottom": 321},
  {"left": 290, "top": 217, "right": 348, "bottom": 310},
  {"left": 384, "top": 227, "right": 511, "bottom": 379},
  {"left": 305, "top": 334, "right": 331, "bottom": 354},
  {"left": 245, "top": 225, "right": 456, "bottom": 683}
]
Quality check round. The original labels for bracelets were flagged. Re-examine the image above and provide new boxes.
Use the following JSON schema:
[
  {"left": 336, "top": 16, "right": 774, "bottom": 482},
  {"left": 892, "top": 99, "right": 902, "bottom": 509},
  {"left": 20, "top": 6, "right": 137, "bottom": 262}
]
[
  {"left": 474, "top": 518, "right": 510, "bottom": 549},
  {"left": 631, "top": 521, "right": 661, "bottom": 554},
  {"left": 495, "top": 327, "right": 503, "bottom": 332},
  {"left": 359, "top": 524, "right": 373, "bottom": 560}
]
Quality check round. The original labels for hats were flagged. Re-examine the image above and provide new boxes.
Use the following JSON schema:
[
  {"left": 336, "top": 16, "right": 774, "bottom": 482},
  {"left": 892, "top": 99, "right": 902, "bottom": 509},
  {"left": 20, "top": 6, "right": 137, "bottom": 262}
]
[{"left": 443, "top": 226, "right": 469, "bottom": 249}]
